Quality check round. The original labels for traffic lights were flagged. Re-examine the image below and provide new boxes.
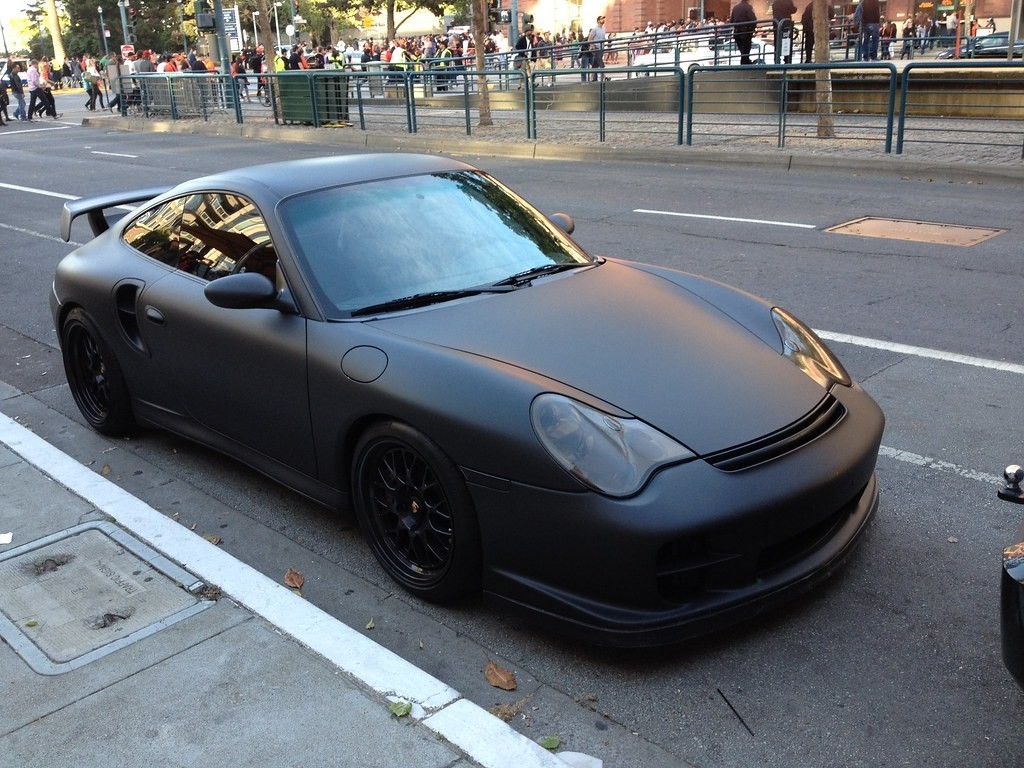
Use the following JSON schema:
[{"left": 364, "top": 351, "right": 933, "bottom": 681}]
[
  {"left": 123, "top": 0, "right": 134, "bottom": 8},
  {"left": 129, "top": 8, "right": 137, "bottom": 27},
  {"left": 294, "top": 2, "right": 300, "bottom": 15},
  {"left": 522, "top": 13, "right": 534, "bottom": 33}
]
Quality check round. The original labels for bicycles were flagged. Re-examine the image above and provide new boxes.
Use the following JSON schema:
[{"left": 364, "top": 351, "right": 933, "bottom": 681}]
[{"left": 257, "top": 85, "right": 272, "bottom": 107}]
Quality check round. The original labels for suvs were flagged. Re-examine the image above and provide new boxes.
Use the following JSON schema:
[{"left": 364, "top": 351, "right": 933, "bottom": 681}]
[{"left": 633, "top": 35, "right": 725, "bottom": 78}]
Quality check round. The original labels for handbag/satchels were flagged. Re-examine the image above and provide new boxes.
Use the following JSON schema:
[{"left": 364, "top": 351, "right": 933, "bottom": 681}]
[
  {"left": 84, "top": 71, "right": 99, "bottom": 84},
  {"left": 513, "top": 57, "right": 526, "bottom": 70},
  {"left": 577, "top": 46, "right": 583, "bottom": 59},
  {"left": 905, "top": 41, "right": 911, "bottom": 48},
  {"left": 883, "top": 37, "right": 890, "bottom": 44}
]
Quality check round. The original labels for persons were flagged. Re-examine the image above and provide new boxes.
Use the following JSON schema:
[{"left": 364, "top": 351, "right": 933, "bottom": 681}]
[{"left": 0, "top": 0, "right": 1000, "bottom": 126}]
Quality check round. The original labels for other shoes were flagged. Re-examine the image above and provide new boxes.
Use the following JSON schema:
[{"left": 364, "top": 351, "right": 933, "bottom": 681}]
[
  {"left": 534, "top": 84, "right": 539, "bottom": 89},
  {"left": 107, "top": 104, "right": 113, "bottom": 113},
  {"left": 0, "top": 121, "right": 8, "bottom": 126},
  {"left": 22, "top": 119, "right": 29, "bottom": 121},
  {"left": 38, "top": 110, "right": 42, "bottom": 118},
  {"left": 33, "top": 111, "right": 37, "bottom": 116},
  {"left": 547, "top": 84, "right": 552, "bottom": 87},
  {"left": 870, "top": 58, "right": 881, "bottom": 62},
  {"left": 6, "top": 119, "right": 13, "bottom": 121},
  {"left": 605, "top": 77, "right": 611, "bottom": 82},
  {"left": 13, "top": 113, "right": 18, "bottom": 119},
  {"left": 54, "top": 113, "right": 63, "bottom": 119}
]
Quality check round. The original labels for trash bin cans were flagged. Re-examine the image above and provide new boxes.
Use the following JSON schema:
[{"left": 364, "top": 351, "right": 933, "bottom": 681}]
[{"left": 276, "top": 69, "right": 350, "bottom": 126}]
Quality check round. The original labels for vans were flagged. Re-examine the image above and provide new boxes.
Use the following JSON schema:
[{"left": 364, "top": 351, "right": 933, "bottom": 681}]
[{"left": 0, "top": 59, "right": 31, "bottom": 88}]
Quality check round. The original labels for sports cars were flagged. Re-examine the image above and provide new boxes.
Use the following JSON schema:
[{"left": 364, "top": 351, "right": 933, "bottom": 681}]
[{"left": 47, "top": 151, "right": 887, "bottom": 651}]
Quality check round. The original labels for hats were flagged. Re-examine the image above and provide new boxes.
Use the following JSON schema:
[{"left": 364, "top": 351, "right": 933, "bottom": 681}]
[
  {"left": 281, "top": 49, "right": 288, "bottom": 52},
  {"left": 987, "top": 18, "right": 993, "bottom": 22},
  {"left": 256, "top": 48, "right": 263, "bottom": 53},
  {"left": 647, "top": 21, "right": 652, "bottom": 25},
  {"left": 128, "top": 52, "right": 135, "bottom": 56},
  {"left": 143, "top": 50, "right": 150, "bottom": 57},
  {"left": 597, "top": 16, "right": 607, "bottom": 21},
  {"left": 166, "top": 54, "right": 174, "bottom": 59}
]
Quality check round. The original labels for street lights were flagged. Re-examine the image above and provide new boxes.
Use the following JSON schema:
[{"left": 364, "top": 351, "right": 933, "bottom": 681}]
[
  {"left": 97, "top": 6, "right": 110, "bottom": 60},
  {"left": 252, "top": 11, "right": 260, "bottom": 51},
  {"left": 273, "top": 2, "right": 282, "bottom": 57}
]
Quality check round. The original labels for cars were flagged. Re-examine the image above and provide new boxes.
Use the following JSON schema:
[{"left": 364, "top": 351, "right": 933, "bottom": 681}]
[
  {"left": 936, "top": 31, "right": 1024, "bottom": 59},
  {"left": 323, "top": 51, "right": 364, "bottom": 71},
  {"left": 274, "top": 44, "right": 295, "bottom": 58},
  {"left": 723, "top": 38, "right": 785, "bottom": 66}
]
